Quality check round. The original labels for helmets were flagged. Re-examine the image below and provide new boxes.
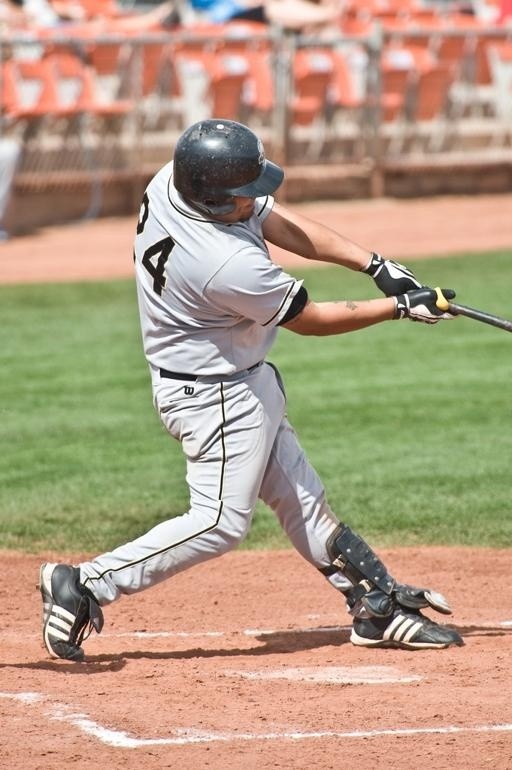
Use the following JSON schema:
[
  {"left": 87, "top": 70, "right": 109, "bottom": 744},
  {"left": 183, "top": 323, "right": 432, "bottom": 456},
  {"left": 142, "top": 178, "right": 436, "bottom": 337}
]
[{"left": 172, "top": 118, "right": 284, "bottom": 216}]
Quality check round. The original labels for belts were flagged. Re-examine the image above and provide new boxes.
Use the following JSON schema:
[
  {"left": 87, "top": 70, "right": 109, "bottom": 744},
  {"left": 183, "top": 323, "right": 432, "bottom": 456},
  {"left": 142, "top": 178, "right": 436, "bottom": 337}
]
[{"left": 160, "top": 368, "right": 196, "bottom": 382}]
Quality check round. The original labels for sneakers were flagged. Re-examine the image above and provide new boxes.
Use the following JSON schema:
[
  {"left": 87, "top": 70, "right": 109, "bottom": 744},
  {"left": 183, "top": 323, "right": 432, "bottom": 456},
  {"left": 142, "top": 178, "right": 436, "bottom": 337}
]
[
  {"left": 351, "top": 611, "right": 455, "bottom": 650},
  {"left": 38, "top": 563, "right": 105, "bottom": 659}
]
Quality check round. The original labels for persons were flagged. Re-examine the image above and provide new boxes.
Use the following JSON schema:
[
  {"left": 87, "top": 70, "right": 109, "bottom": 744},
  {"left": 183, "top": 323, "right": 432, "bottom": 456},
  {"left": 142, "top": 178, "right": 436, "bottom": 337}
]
[{"left": 39, "top": 116, "right": 462, "bottom": 663}]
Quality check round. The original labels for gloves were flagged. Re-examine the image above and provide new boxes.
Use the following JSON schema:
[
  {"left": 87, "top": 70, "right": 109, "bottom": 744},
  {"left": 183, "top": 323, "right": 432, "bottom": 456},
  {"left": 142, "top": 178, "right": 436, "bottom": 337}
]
[
  {"left": 392, "top": 288, "right": 456, "bottom": 325},
  {"left": 360, "top": 251, "right": 423, "bottom": 297}
]
